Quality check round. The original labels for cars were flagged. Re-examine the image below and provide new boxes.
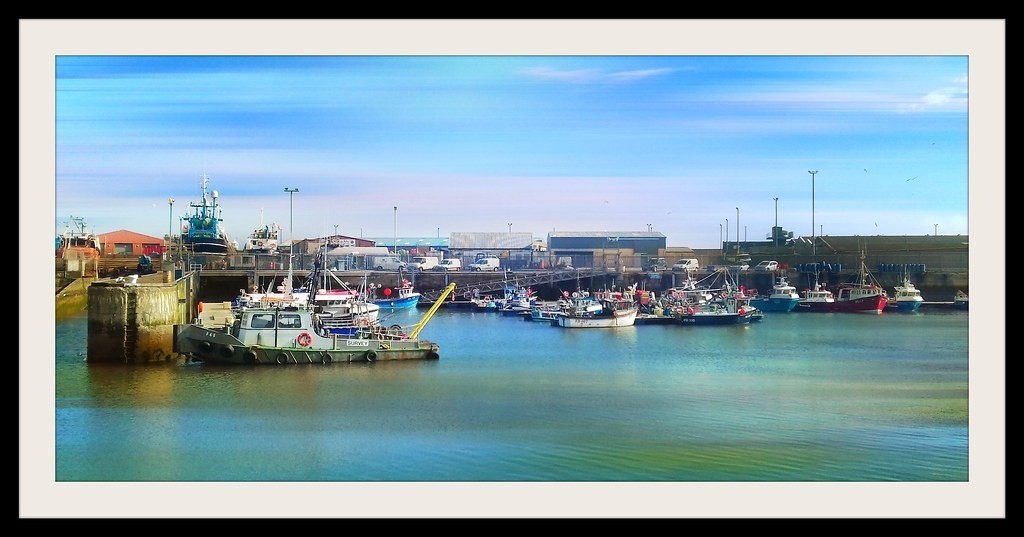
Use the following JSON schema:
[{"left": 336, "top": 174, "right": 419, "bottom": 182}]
[{"left": 755, "top": 260, "right": 778, "bottom": 272}]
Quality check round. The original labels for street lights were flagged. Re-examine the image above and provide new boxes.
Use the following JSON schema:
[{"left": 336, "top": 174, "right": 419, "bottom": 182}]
[
  {"left": 808, "top": 170, "right": 819, "bottom": 261},
  {"left": 283, "top": 187, "right": 299, "bottom": 268},
  {"left": 736, "top": 207, "right": 740, "bottom": 254},
  {"left": 720, "top": 224, "right": 722, "bottom": 256},
  {"left": 772, "top": 197, "right": 778, "bottom": 249},
  {"left": 393, "top": 206, "right": 397, "bottom": 260},
  {"left": 725, "top": 219, "right": 728, "bottom": 253}
]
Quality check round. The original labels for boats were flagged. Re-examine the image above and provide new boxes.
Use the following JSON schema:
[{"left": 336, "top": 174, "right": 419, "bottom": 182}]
[
  {"left": 748, "top": 275, "right": 798, "bottom": 314},
  {"left": 832, "top": 238, "right": 888, "bottom": 315},
  {"left": 469, "top": 263, "right": 765, "bottom": 329},
  {"left": 953, "top": 289, "right": 969, "bottom": 310},
  {"left": 795, "top": 261, "right": 835, "bottom": 313},
  {"left": 883, "top": 265, "right": 925, "bottom": 312},
  {"left": 246, "top": 224, "right": 280, "bottom": 253},
  {"left": 172, "top": 234, "right": 457, "bottom": 365},
  {"left": 177, "top": 171, "right": 227, "bottom": 253},
  {"left": 365, "top": 278, "right": 422, "bottom": 311}
]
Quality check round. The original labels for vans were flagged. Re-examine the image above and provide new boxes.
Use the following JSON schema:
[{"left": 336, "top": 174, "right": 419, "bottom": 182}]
[
  {"left": 467, "top": 257, "right": 500, "bottom": 272},
  {"left": 432, "top": 257, "right": 462, "bottom": 271},
  {"left": 373, "top": 257, "right": 405, "bottom": 272},
  {"left": 672, "top": 258, "right": 699, "bottom": 272},
  {"left": 406, "top": 255, "right": 439, "bottom": 272}
]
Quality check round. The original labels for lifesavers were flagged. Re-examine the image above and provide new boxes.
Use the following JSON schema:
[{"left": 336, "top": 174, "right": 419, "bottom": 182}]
[
  {"left": 366, "top": 349, "right": 378, "bottom": 363},
  {"left": 219, "top": 344, "right": 235, "bottom": 358},
  {"left": 199, "top": 341, "right": 211, "bottom": 354},
  {"left": 675, "top": 306, "right": 684, "bottom": 315},
  {"left": 258, "top": 241, "right": 263, "bottom": 246},
  {"left": 298, "top": 332, "right": 312, "bottom": 347},
  {"left": 597, "top": 292, "right": 604, "bottom": 301},
  {"left": 244, "top": 350, "right": 257, "bottom": 364},
  {"left": 277, "top": 352, "right": 289, "bottom": 365},
  {"left": 484, "top": 297, "right": 490, "bottom": 303},
  {"left": 321, "top": 353, "right": 333, "bottom": 365}
]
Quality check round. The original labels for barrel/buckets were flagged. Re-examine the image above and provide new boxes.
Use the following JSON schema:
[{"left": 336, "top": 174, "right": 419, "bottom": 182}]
[
  {"left": 163, "top": 270, "right": 171, "bottom": 283},
  {"left": 796, "top": 260, "right": 841, "bottom": 272},
  {"left": 879, "top": 263, "right": 925, "bottom": 272},
  {"left": 176, "top": 270, "right": 182, "bottom": 280}
]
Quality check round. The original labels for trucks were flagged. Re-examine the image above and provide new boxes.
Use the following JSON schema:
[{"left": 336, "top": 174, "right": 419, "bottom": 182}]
[{"left": 724, "top": 253, "right": 752, "bottom": 265}]
